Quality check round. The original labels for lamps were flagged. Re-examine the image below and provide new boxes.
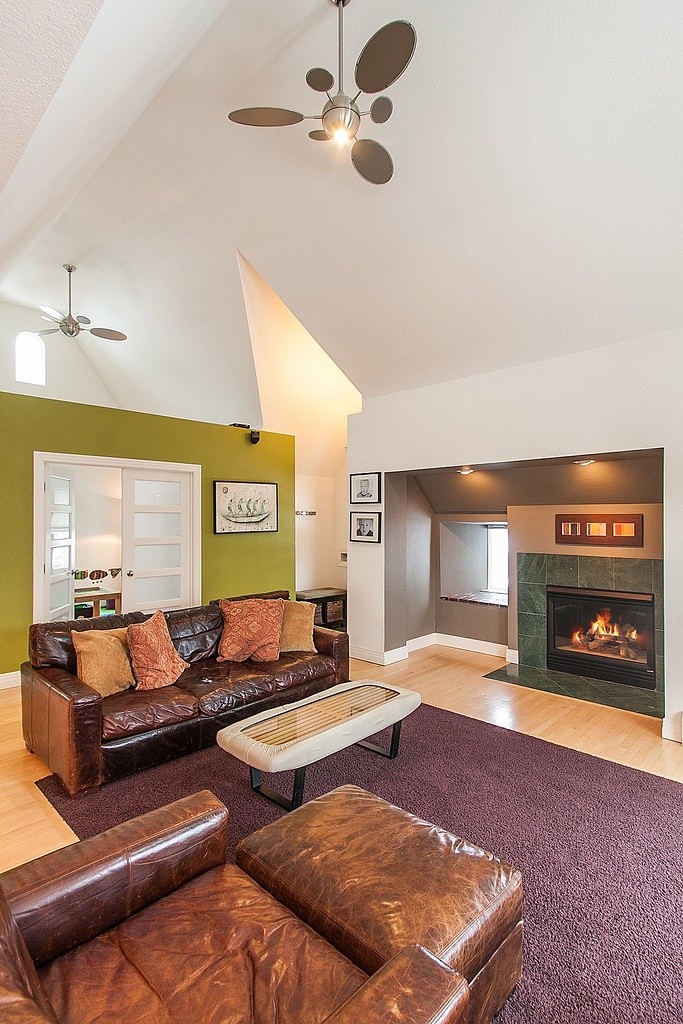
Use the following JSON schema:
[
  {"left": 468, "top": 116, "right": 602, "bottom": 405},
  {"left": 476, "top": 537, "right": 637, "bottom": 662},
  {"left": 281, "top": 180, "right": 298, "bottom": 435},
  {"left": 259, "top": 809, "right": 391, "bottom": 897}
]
[
  {"left": 321, "top": 105, "right": 360, "bottom": 141},
  {"left": 59, "top": 326, "right": 80, "bottom": 337}
]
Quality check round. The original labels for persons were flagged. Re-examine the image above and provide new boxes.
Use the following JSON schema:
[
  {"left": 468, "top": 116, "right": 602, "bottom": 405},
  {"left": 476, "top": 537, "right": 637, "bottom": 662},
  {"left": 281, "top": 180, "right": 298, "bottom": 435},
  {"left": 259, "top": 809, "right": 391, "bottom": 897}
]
[
  {"left": 357, "top": 479, "right": 372, "bottom": 497},
  {"left": 357, "top": 518, "right": 373, "bottom": 536}
]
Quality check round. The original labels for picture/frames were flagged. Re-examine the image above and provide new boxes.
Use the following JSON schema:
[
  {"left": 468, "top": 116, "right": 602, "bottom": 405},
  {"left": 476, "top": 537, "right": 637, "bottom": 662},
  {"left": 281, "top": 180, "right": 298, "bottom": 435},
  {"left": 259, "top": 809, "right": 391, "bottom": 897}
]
[
  {"left": 350, "top": 472, "right": 381, "bottom": 504},
  {"left": 350, "top": 511, "right": 381, "bottom": 543},
  {"left": 213, "top": 480, "right": 278, "bottom": 534}
]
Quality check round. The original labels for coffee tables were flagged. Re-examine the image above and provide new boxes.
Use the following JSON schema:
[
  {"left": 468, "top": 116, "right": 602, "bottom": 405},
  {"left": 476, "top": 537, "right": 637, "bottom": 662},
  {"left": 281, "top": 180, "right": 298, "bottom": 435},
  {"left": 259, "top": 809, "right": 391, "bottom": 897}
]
[{"left": 216, "top": 679, "right": 422, "bottom": 811}]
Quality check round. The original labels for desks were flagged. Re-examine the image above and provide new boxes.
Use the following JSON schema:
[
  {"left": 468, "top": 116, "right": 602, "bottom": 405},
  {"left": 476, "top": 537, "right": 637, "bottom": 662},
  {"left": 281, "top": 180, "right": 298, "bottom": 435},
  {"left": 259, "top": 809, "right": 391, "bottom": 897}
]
[
  {"left": 295, "top": 587, "right": 346, "bottom": 625},
  {"left": 75, "top": 589, "right": 121, "bottom": 617}
]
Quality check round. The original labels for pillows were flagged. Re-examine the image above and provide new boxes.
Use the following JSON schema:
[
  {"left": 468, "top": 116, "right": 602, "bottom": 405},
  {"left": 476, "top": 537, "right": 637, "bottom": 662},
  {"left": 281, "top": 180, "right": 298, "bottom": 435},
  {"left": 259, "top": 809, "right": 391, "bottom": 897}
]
[
  {"left": 280, "top": 599, "right": 318, "bottom": 653},
  {"left": 127, "top": 609, "right": 191, "bottom": 691},
  {"left": 216, "top": 598, "right": 284, "bottom": 662},
  {"left": 71, "top": 628, "right": 137, "bottom": 698}
]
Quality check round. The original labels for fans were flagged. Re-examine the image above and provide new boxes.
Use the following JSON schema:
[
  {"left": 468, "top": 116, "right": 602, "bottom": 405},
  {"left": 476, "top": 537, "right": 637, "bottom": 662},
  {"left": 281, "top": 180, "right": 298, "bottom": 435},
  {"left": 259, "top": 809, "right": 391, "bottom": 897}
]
[
  {"left": 228, "top": 0, "right": 418, "bottom": 185},
  {"left": 33, "top": 264, "right": 127, "bottom": 341}
]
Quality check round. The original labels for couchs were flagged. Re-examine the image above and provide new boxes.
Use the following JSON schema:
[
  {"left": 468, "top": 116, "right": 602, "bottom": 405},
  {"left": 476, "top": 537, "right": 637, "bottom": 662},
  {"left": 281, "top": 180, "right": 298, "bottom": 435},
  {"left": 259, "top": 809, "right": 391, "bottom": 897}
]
[
  {"left": 19, "top": 590, "right": 349, "bottom": 795},
  {"left": 0, "top": 790, "right": 471, "bottom": 1024}
]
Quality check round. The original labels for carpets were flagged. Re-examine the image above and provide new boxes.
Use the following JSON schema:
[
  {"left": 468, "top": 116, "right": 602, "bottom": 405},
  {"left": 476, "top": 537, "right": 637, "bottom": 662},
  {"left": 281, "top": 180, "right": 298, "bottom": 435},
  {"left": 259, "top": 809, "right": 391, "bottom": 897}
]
[{"left": 36, "top": 686, "right": 683, "bottom": 1024}]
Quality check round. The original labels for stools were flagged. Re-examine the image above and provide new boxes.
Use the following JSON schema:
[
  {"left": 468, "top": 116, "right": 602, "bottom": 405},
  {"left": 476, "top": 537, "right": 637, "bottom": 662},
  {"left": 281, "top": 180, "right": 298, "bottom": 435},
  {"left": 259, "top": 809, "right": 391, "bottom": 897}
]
[{"left": 234, "top": 784, "right": 523, "bottom": 1024}]
